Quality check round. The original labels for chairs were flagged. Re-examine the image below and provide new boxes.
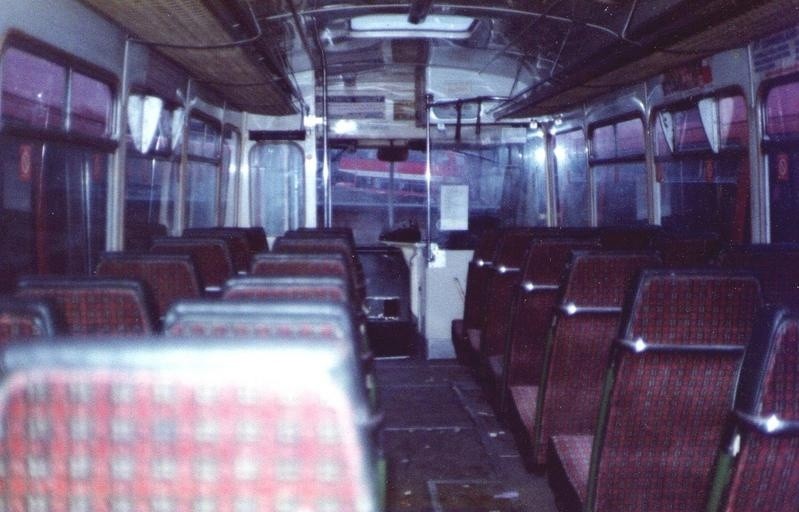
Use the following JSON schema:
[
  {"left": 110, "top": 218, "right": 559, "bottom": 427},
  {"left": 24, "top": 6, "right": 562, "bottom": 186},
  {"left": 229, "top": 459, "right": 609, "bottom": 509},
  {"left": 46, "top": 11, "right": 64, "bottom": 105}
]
[
  {"left": 246, "top": 227, "right": 270, "bottom": 255},
  {"left": 146, "top": 236, "right": 238, "bottom": 300},
  {"left": 9, "top": 276, "right": 158, "bottom": 335},
  {"left": 270, "top": 236, "right": 365, "bottom": 299},
  {"left": 0, "top": 338, "right": 377, "bottom": 511},
  {"left": 159, "top": 301, "right": 358, "bottom": 339},
  {"left": 284, "top": 228, "right": 353, "bottom": 237},
  {"left": 644, "top": 231, "right": 724, "bottom": 272},
  {"left": 93, "top": 252, "right": 202, "bottom": 325},
  {"left": 704, "top": 305, "right": 799, "bottom": 512},
  {"left": 507, "top": 252, "right": 666, "bottom": 465},
  {"left": 488, "top": 239, "right": 612, "bottom": 413},
  {"left": 593, "top": 225, "right": 664, "bottom": 251},
  {"left": 219, "top": 274, "right": 355, "bottom": 305},
  {"left": 717, "top": 244, "right": 798, "bottom": 306},
  {"left": 180, "top": 228, "right": 255, "bottom": 274},
  {"left": 1, "top": 299, "right": 64, "bottom": 337},
  {"left": 550, "top": 272, "right": 759, "bottom": 512},
  {"left": 453, "top": 230, "right": 535, "bottom": 366},
  {"left": 247, "top": 254, "right": 353, "bottom": 276}
]
